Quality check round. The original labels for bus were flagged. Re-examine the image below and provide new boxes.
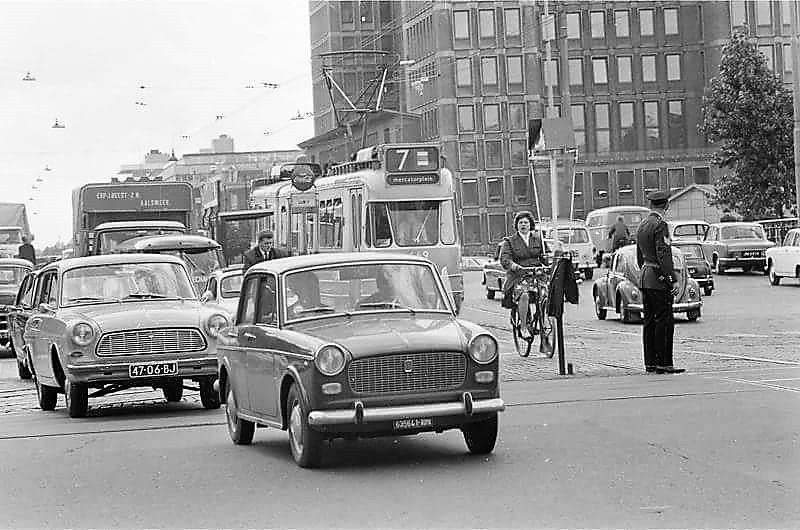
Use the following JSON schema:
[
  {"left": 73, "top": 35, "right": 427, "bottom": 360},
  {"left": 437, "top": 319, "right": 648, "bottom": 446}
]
[
  {"left": 68, "top": 180, "right": 195, "bottom": 257},
  {"left": 245, "top": 48, "right": 465, "bottom": 322}
]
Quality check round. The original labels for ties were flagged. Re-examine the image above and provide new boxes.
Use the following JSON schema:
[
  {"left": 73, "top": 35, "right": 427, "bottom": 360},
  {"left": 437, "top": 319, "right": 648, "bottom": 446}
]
[{"left": 265, "top": 251, "right": 269, "bottom": 260}]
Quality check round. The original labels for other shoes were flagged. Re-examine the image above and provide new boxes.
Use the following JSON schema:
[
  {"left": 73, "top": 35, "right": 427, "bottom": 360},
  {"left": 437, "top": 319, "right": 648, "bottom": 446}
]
[
  {"left": 646, "top": 367, "right": 656, "bottom": 372},
  {"left": 521, "top": 328, "right": 532, "bottom": 339},
  {"left": 539, "top": 336, "right": 553, "bottom": 352},
  {"left": 656, "top": 366, "right": 685, "bottom": 374}
]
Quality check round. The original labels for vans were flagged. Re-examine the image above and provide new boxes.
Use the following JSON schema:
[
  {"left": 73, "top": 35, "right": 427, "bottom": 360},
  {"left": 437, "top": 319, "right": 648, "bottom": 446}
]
[{"left": 584, "top": 205, "right": 652, "bottom": 269}]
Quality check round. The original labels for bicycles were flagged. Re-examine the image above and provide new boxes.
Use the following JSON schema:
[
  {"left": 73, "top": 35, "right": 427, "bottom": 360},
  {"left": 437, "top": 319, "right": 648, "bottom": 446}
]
[{"left": 507, "top": 264, "right": 558, "bottom": 358}]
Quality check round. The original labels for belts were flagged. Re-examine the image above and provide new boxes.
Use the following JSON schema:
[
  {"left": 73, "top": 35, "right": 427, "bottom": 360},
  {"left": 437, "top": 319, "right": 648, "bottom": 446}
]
[{"left": 644, "top": 259, "right": 660, "bottom": 268}]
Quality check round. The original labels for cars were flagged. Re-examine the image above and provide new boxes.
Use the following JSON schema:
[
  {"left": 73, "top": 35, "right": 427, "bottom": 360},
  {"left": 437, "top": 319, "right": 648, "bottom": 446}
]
[
  {"left": 591, "top": 244, "right": 705, "bottom": 325},
  {"left": 481, "top": 236, "right": 584, "bottom": 307},
  {"left": 667, "top": 220, "right": 713, "bottom": 245},
  {"left": 704, "top": 221, "right": 778, "bottom": 279},
  {"left": 214, "top": 248, "right": 507, "bottom": 469},
  {"left": 670, "top": 240, "right": 715, "bottom": 297},
  {"left": 540, "top": 219, "right": 599, "bottom": 282},
  {"left": 762, "top": 228, "right": 800, "bottom": 287},
  {"left": 0, "top": 221, "right": 297, "bottom": 418}
]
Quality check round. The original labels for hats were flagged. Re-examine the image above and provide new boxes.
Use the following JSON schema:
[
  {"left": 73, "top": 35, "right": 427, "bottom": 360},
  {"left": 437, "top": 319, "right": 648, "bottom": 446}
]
[
  {"left": 647, "top": 192, "right": 672, "bottom": 203},
  {"left": 259, "top": 230, "right": 273, "bottom": 241}
]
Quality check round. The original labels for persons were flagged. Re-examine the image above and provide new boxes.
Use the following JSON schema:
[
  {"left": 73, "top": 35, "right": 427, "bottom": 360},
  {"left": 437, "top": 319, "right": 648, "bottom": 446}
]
[
  {"left": 287, "top": 271, "right": 333, "bottom": 319},
  {"left": 133, "top": 269, "right": 161, "bottom": 293},
  {"left": 608, "top": 216, "right": 630, "bottom": 249},
  {"left": 500, "top": 212, "right": 554, "bottom": 353},
  {"left": 20, "top": 235, "right": 37, "bottom": 265},
  {"left": 357, "top": 266, "right": 423, "bottom": 311},
  {"left": 636, "top": 192, "right": 686, "bottom": 374},
  {"left": 719, "top": 208, "right": 737, "bottom": 223},
  {"left": 399, "top": 209, "right": 427, "bottom": 244},
  {"left": 241, "top": 229, "right": 292, "bottom": 312}
]
